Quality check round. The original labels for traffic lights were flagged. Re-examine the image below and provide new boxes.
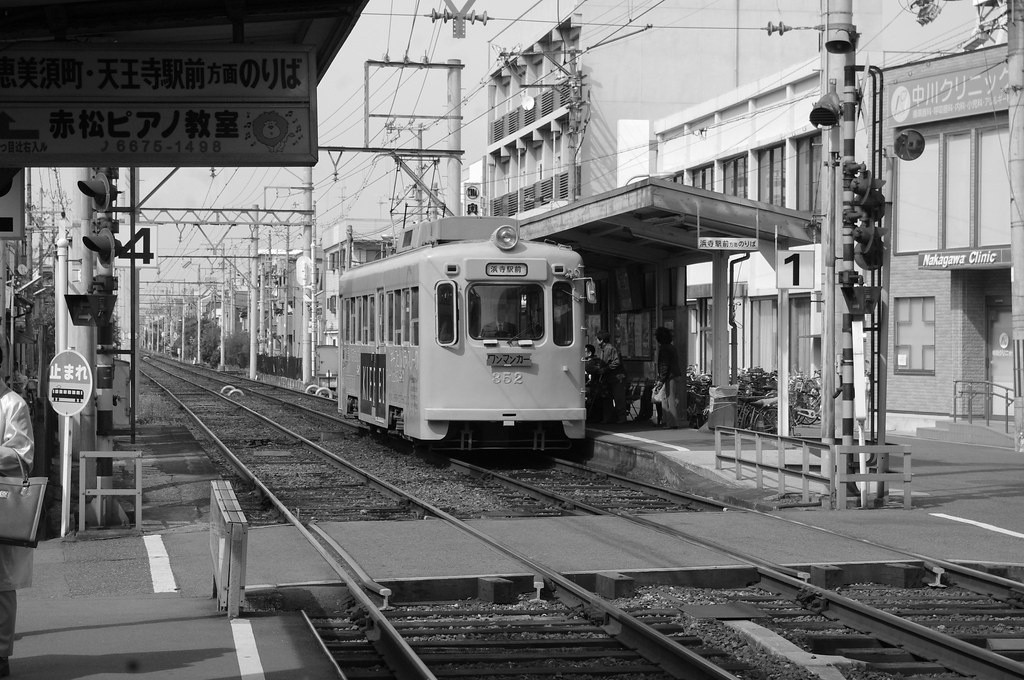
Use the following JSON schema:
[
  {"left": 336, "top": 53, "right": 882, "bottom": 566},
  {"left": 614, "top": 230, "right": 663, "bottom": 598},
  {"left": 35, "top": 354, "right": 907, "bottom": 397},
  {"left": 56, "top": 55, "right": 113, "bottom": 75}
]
[
  {"left": 841, "top": 285, "right": 885, "bottom": 315},
  {"left": 849, "top": 169, "right": 887, "bottom": 207},
  {"left": 851, "top": 215, "right": 891, "bottom": 257},
  {"left": 82, "top": 228, "right": 122, "bottom": 269},
  {"left": 62, "top": 292, "right": 118, "bottom": 328},
  {"left": 77, "top": 171, "right": 118, "bottom": 213},
  {"left": 809, "top": 91, "right": 845, "bottom": 128}
]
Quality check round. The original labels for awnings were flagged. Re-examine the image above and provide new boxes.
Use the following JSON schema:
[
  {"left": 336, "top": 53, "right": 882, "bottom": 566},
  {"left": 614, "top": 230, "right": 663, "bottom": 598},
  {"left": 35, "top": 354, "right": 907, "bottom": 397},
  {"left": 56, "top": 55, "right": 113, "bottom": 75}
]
[{"left": 15, "top": 330, "right": 37, "bottom": 343}]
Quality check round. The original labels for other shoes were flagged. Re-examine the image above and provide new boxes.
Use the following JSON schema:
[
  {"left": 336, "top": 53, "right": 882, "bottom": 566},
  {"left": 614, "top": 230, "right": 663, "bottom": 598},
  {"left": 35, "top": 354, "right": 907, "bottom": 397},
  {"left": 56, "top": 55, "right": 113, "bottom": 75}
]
[{"left": 0, "top": 657, "right": 10, "bottom": 677}]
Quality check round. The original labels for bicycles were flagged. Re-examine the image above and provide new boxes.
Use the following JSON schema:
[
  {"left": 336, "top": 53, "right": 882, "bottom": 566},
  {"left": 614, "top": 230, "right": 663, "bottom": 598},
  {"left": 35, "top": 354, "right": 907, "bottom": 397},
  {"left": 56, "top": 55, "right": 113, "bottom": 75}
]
[{"left": 687, "top": 362, "right": 823, "bottom": 436}]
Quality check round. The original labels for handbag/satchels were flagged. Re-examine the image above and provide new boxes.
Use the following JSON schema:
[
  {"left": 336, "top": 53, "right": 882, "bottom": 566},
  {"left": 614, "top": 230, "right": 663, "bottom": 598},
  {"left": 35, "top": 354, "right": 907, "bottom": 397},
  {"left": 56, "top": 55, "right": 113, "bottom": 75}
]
[
  {"left": 651, "top": 383, "right": 666, "bottom": 403},
  {"left": 0, "top": 447, "right": 48, "bottom": 542}
]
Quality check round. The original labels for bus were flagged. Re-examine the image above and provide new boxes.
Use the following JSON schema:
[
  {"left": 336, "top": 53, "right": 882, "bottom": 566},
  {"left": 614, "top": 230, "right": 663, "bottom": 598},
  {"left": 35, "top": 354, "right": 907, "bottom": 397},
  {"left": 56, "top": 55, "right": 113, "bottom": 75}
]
[{"left": 335, "top": 213, "right": 597, "bottom": 456}]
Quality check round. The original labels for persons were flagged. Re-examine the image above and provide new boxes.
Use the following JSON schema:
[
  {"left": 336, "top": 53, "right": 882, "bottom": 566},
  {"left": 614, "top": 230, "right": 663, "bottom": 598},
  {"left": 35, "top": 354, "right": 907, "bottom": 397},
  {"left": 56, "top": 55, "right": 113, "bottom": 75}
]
[
  {"left": 478, "top": 304, "right": 517, "bottom": 338},
  {"left": 0, "top": 346, "right": 34, "bottom": 677},
  {"left": 655, "top": 327, "right": 687, "bottom": 429},
  {"left": 597, "top": 330, "right": 627, "bottom": 423},
  {"left": 585, "top": 345, "right": 602, "bottom": 417},
  {"left": 6, "top": 370, "right": 34, "bottom": 404}
]
